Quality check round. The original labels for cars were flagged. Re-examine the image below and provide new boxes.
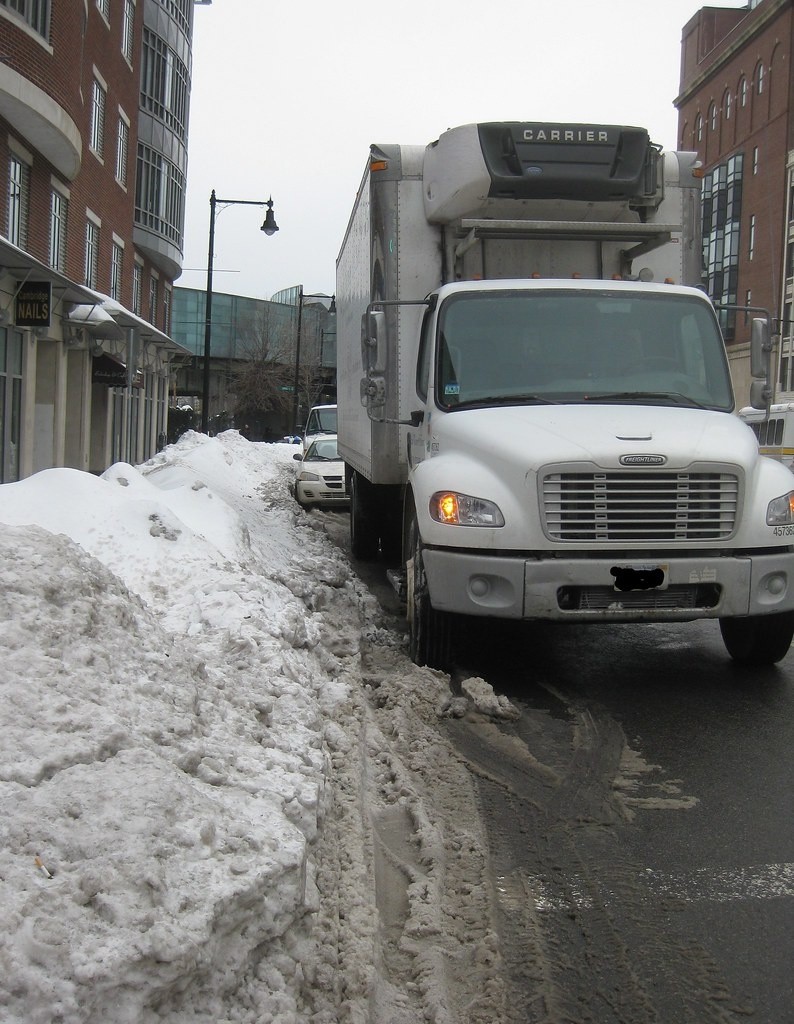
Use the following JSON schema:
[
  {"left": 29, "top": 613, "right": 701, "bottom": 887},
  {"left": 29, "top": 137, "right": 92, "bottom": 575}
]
[{"left": 293, "top": 436, "right": 351, "bottom": 508}]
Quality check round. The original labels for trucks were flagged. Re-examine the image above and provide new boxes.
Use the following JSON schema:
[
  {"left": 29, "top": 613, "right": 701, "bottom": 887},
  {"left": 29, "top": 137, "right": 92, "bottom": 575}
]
[
  {"left": 334, "top": 120, "right": 794, "bottom": 674},
  {"left": 737, "top": 403, "right": 794, "bottom": 475}
]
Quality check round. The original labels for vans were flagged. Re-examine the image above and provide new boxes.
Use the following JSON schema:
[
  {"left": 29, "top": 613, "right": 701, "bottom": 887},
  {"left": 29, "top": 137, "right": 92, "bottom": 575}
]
[{"left": 296, "top": 405, "right": 338, "bottom": 455}]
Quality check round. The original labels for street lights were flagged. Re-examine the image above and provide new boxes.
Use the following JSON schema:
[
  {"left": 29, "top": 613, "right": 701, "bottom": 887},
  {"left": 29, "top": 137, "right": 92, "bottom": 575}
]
[
  {"left": 201, "top": 189, "right": 280, "bottom": 435},
  {"left": 290, "top": 289, "right": 337, "bottom": 435},
  {"left": 770, "top": 318, "right": 794, "bottom": 345}
]
